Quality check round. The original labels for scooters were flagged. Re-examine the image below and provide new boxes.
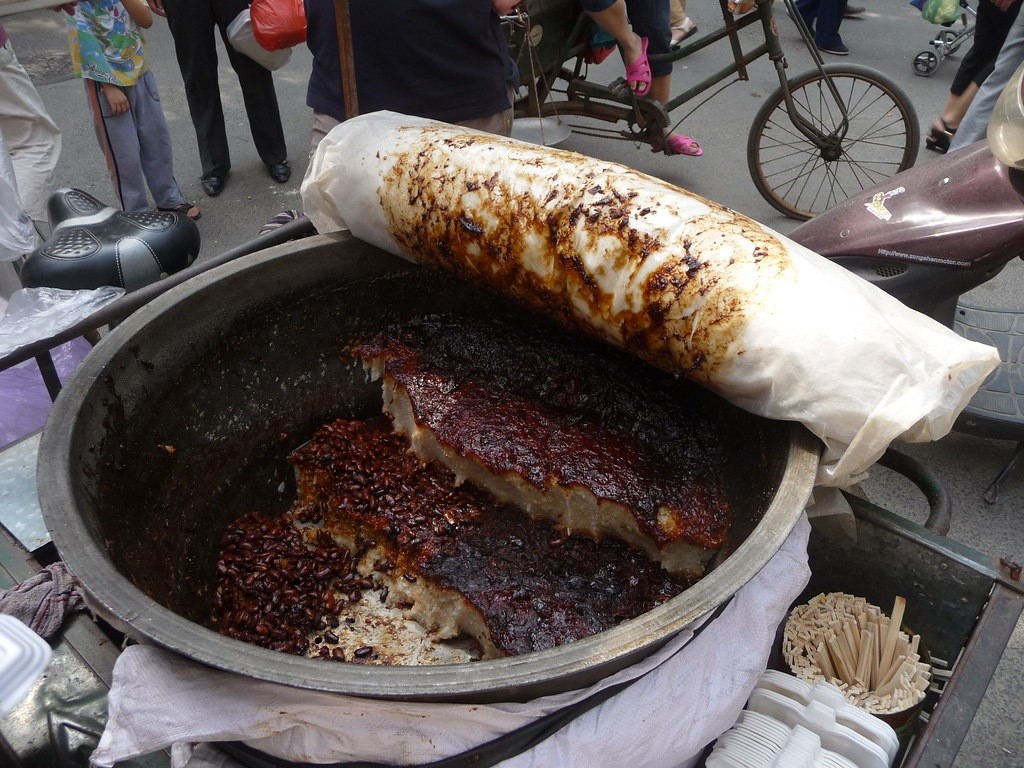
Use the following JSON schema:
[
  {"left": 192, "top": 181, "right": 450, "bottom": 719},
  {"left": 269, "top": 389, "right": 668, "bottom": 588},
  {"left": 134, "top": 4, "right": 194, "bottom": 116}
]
[{"left": 784, "top": 59, "right": 1024, "bottom": 504}]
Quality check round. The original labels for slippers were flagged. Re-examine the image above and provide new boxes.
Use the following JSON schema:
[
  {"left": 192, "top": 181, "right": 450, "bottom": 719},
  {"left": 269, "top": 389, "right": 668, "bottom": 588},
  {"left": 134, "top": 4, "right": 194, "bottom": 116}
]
[
  {"left": 624, "top": 36, "right": 651, "bottom": 96},
  {"left": 652, "top": 134, "right": 703, "bottom": 156}
]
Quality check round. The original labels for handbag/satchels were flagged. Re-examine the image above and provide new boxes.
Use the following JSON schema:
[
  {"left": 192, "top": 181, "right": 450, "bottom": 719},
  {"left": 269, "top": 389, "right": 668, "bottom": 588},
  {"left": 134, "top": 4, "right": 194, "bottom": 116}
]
[
  {"left": 250, "top": 0, "right": 311, "bottom": 53},
  {"left": 227, "top": 7, "right": 293, "bottom": 71}
]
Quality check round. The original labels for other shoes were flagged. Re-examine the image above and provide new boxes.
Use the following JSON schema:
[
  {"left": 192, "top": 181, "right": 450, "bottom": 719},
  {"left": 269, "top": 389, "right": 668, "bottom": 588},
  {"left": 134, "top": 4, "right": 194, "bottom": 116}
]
[
  {"left": 787, "top": 9, "right": 816, "bottom": 38},
  {"left": 844, "top": 3, "right": 865, "bottom": 14},
  {"left": 817, "top": 44, "right": 850, "bottom": 55}
]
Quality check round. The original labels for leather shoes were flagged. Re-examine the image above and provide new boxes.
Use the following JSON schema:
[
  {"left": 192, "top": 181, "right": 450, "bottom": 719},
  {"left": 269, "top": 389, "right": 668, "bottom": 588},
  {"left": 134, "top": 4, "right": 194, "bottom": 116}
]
[
  {"left": 269, "top": 162, "right": 290, "bottom": 183},
  {"left": 202, "top": 173, "right": 223, "bottom": 195}
]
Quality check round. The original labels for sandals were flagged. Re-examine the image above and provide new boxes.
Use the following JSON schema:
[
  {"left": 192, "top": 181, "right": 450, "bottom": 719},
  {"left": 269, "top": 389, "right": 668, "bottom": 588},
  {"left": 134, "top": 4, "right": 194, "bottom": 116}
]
[
  {"left": 158, "top": 204, "right": 201, "bottom": 220},
  {"left": 926, "top": 118, "right": 956, "bottom": 152},
  {"left": 669, "top": 15, "right": 697, "bottom": 48}
]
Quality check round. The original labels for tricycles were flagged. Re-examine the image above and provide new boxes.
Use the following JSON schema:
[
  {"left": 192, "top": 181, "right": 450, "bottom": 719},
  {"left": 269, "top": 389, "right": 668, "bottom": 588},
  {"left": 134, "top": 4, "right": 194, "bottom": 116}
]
[
  {"left": 0, "top": 189, "right": 1024, "bottom": 768},
  {"left": 500, "top": 0, "right": 920, "bottom": 221}
]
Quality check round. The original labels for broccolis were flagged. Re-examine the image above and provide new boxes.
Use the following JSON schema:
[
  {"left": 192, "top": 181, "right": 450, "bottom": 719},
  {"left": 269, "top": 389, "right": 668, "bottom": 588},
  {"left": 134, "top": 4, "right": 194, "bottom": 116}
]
[{"left": 925, "top": 0, "right": 958, "bottom": 23}]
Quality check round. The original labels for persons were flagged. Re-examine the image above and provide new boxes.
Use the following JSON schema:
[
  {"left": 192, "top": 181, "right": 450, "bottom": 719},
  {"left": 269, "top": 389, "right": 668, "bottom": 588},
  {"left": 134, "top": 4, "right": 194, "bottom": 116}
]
[
  {"left": 580, "top": 0, "right": 703, "bottom": 156},
  {"left": 669, "top": 0, "right": 699, "bottom": 48},
  {"left": 146, "top": 0, "right": 305, "bottom": 196},
  {"left": 785, "top": 0, "right": 866, "bottom": 55},
  {"left": 0, "top": 2, "right": 79, "bottom": 223},
  {"left": 924, "top": 0, "right": 1024, "bottom": 264},
  {"left": 64, "top": 0, "right": 203, "bottom": 221},
  {"left": 303, "top": 0, "right": 521, "bottom": 166}
]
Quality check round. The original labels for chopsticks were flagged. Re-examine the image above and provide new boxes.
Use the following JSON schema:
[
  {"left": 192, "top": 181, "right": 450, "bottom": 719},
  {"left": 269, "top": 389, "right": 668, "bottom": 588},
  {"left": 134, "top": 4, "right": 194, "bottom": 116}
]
[{"left": 781, "top": 591, "right": 932, "bottom": 715}]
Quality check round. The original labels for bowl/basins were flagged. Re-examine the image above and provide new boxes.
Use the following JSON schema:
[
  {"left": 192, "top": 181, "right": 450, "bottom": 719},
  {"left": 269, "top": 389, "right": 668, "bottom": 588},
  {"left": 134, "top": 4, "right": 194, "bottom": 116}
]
[{"left": 38, "top": 232, "right": 820, "bottom": 700}]
